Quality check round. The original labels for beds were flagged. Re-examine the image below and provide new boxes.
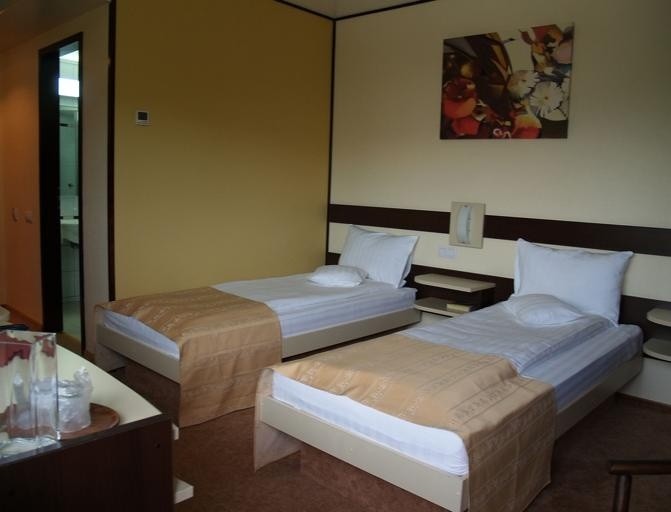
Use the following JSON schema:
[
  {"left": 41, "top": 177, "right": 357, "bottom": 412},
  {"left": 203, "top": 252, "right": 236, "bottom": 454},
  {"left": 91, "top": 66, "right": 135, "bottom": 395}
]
[
  {"left": 93, "top": 266, "right": 437, "bottom": 429},
  {"left": 254, "top": 298, "right": 644, "bottom": 511}
]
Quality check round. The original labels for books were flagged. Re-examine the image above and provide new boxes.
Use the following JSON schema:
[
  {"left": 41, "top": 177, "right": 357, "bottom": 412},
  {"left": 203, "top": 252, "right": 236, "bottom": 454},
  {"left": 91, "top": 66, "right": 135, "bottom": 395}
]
[{"left": 446, "top": 303, "right": 482, "bottom": 313}]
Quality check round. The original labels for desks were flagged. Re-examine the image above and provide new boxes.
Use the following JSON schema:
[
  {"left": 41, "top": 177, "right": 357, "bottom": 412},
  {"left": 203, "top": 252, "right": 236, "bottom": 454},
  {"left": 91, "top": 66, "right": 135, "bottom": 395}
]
[{"left": 0, "top": 303, "right": 194, "bottom": 510}]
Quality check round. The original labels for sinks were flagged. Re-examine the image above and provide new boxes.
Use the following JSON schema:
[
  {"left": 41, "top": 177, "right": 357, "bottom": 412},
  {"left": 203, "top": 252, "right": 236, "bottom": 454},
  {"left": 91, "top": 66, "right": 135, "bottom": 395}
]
[{"left": 60, "top": 219, "right": 79, "bottom": 244}]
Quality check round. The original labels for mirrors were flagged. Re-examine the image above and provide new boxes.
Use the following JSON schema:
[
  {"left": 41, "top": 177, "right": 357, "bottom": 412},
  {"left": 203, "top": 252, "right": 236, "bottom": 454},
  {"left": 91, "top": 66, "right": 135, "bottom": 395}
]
[{"left": 60, "top": 109, "right": 79, "bottom": 197}]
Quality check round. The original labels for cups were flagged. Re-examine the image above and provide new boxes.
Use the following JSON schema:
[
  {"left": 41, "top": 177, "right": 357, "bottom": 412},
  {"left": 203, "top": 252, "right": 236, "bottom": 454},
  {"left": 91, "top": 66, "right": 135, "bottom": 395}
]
[{"left": 8, "top": 373, "right": 98, "bottom": 437}]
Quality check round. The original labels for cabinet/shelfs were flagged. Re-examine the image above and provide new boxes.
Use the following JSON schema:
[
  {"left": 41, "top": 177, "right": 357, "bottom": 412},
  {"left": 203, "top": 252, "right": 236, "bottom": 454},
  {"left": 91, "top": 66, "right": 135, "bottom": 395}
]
[{"left": 642, "top": 309, "right": 671, "bottom": 362}]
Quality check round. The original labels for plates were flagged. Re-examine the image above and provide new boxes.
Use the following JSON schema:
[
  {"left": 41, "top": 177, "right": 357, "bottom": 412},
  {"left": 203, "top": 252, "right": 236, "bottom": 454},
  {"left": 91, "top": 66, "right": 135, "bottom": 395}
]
[{"left": 2, "top": 399, "right": 121, "bottom": 442}]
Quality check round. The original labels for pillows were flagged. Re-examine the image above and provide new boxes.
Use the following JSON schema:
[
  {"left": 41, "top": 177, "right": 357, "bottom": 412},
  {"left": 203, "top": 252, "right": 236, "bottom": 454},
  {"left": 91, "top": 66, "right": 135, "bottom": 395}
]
[
  {"left": 306, "top": 224, "right": 422, "bottom": 288},
  {"left": 503, "top": 234, "right": 636, "bottom": 326}
]
